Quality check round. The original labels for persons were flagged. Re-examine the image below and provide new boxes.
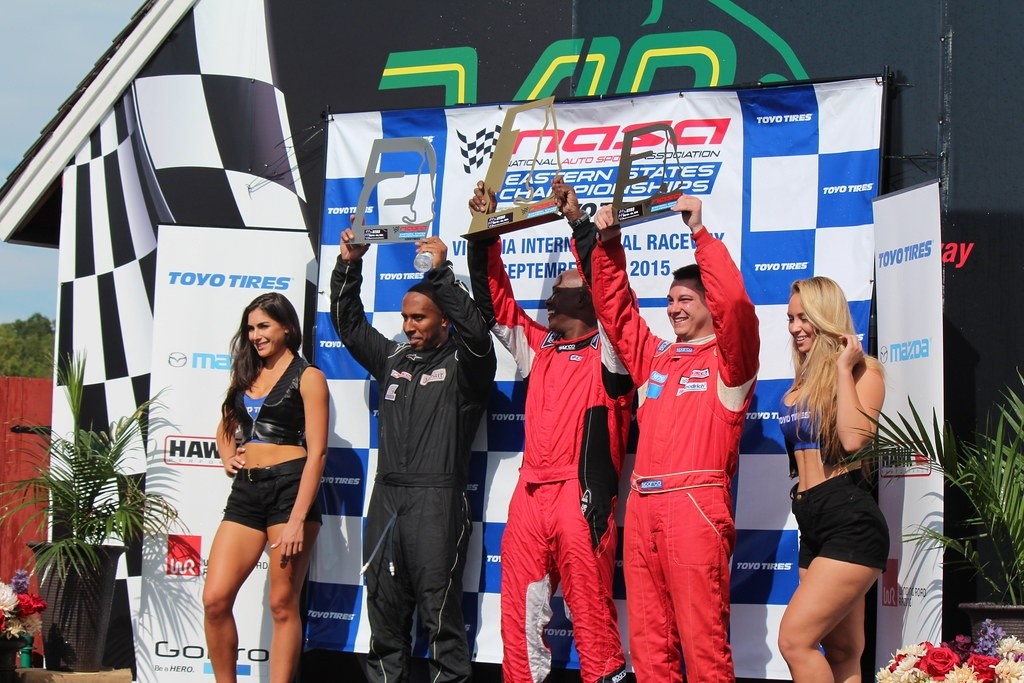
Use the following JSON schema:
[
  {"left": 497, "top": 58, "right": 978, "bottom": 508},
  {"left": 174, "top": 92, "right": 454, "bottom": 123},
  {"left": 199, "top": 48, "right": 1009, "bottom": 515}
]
[
  {"left": 326, "top": 211, "right": 498, "bottom": 683},
  {"left": 772, "top": 275, "right": 891, "bottom": 683},
  {"left": 594, "top": 193, "right": 761, "bottom": 678},
  {"left": 200, "top": 291, "right": 336, "bottom": 683},
  {"left": 468, "top": 173, "right": 638, "bottom": 683}
]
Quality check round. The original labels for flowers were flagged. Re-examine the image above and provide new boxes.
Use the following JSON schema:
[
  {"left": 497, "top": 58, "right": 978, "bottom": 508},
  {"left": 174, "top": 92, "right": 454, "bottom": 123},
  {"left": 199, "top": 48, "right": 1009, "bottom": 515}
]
[
  {"left": 872, "top": 619, "right": 1024, "bottom": 683},
  {"left": 0, "top": 567, "right": 48, "bottom": 643}
]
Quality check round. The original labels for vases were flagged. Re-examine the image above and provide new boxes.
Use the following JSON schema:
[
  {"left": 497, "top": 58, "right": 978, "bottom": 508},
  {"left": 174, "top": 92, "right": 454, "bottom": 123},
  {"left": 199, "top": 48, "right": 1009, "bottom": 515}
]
[{"left": 0, "top": 633, "right": 30, "bottom": 683}]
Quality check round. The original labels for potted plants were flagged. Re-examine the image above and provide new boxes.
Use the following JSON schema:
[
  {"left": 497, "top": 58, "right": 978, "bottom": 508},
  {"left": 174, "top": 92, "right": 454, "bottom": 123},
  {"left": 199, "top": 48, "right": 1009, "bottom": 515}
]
[
  {"left": 828, "top": 366, "right": 1024, "bottom": 644},
  {"left": 5, "top": 348, "right": 179, "bottom": 673}
]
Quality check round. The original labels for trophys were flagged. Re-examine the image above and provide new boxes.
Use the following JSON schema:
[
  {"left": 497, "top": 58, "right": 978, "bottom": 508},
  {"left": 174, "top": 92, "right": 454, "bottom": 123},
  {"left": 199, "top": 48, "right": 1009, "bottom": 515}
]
[
  {"left": 599, "top": 121, "right": 696, "bottom": 236},
  {"left": 342, "top": 134, "right": 440, "bottom": 254},
  {"left": 461, "top": 95, "right": 572, "bottom": 249}
]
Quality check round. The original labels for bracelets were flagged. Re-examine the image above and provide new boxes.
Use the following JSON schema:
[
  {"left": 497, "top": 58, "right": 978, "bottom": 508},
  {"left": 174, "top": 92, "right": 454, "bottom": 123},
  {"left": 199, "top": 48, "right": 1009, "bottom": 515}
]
[{"left": 567, "top": 210, "right": 590, "bottom": 229}]
